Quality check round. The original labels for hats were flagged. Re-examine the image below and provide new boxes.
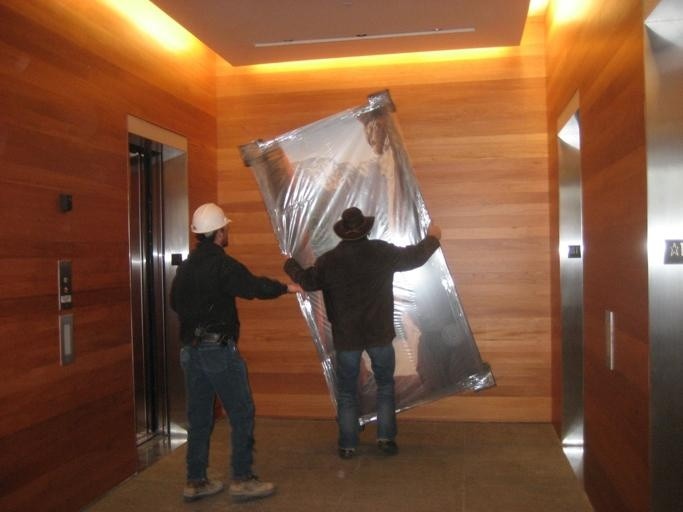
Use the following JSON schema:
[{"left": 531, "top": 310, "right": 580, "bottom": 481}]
[{"left": 333, "top": 208, "right": 374, "bottom": 239}]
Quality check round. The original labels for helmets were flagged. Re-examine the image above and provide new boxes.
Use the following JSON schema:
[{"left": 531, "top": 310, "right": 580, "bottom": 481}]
[{"left": 192, "top": 203, "right": 231, "bottom": 233}]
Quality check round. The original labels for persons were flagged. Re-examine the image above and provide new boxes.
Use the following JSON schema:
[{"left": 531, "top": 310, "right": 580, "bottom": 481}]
[
  {"left": 282, "top": 206, "right": 442, "bottom": 459},
  {"left": 167, "top": 202, "right": 305, "bottom": 503}
]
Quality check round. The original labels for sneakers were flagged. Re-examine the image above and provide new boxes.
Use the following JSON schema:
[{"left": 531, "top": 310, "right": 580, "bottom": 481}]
[
  {"left": 337, "top": 448, "right": 352, "bottom": 459},
  {"left": 230, "top": 476, "right": 274, "bottom": 501},
  {"left": 377, "top": 440, "right": 398, "bottom": 455},
  {"left": 183, "top": 480, "right": 224, "bottom": 500}
]
